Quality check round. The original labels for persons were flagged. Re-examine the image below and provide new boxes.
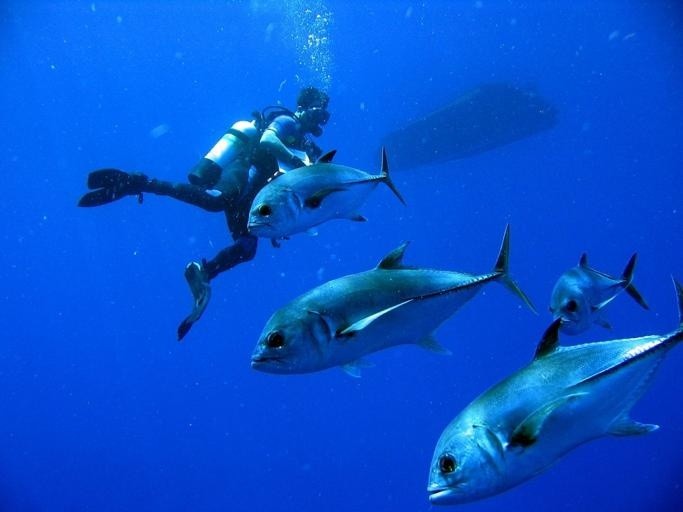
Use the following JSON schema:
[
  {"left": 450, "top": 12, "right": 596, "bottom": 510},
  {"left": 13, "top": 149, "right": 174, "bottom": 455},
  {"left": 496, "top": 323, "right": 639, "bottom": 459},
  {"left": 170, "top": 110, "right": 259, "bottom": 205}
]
[{"left": 78, "top": 88, "right": 330, "bottom": 342}]
